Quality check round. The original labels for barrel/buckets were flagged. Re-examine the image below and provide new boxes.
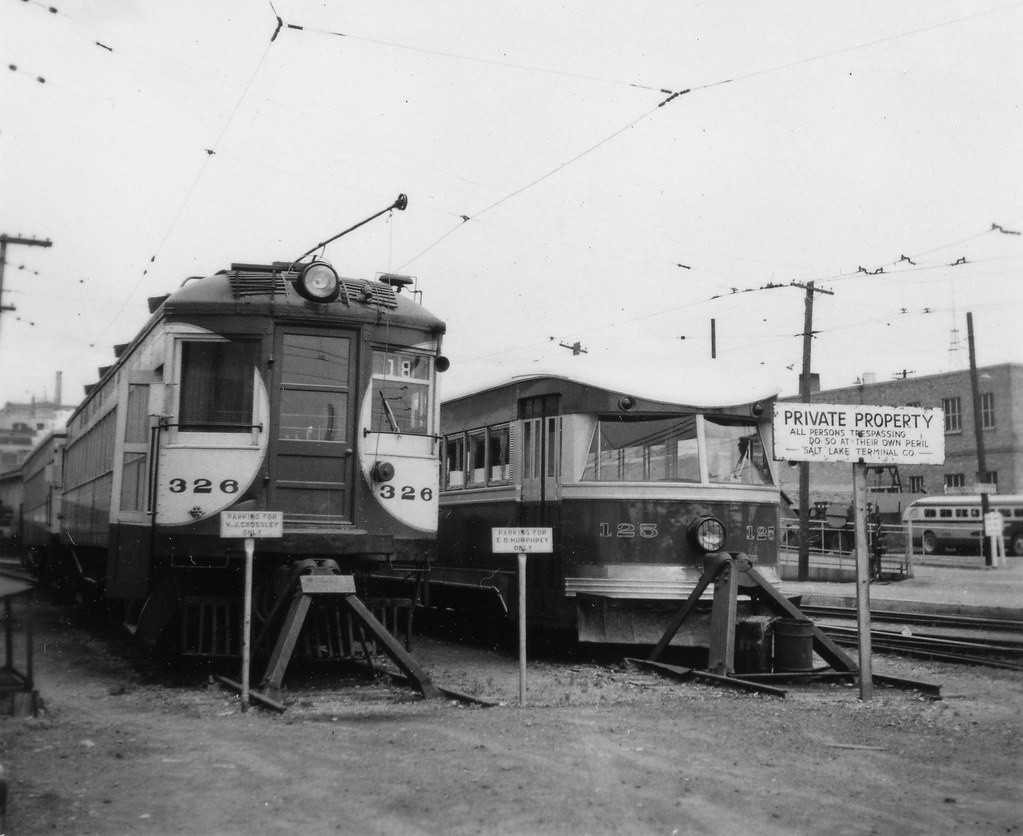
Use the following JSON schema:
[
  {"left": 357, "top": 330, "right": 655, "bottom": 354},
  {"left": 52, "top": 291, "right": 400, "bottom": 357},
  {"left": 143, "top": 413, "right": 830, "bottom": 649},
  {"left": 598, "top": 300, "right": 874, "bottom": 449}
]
[
  {"left": 772, "top": 617, "right": 814, "bottom": 672},
  {"left": 734, "top": 621, "right": 772, "bottom": 674}
]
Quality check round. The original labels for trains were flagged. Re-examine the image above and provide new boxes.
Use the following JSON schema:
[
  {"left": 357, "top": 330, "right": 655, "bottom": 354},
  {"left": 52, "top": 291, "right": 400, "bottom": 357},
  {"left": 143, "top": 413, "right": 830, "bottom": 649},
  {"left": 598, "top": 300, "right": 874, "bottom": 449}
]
[
  {"left": 420, "top": 371, "right": 802, "bottom": 670},
  {"left": 2, "top": 193, "right": 450, "bottom": 681}
]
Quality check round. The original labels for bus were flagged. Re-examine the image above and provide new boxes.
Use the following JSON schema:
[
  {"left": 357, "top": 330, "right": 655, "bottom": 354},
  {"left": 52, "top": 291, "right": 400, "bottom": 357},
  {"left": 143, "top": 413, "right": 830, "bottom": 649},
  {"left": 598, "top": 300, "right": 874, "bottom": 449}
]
[{"left": 899, "top": 493, "right": 1023, "bottom": 556}]
